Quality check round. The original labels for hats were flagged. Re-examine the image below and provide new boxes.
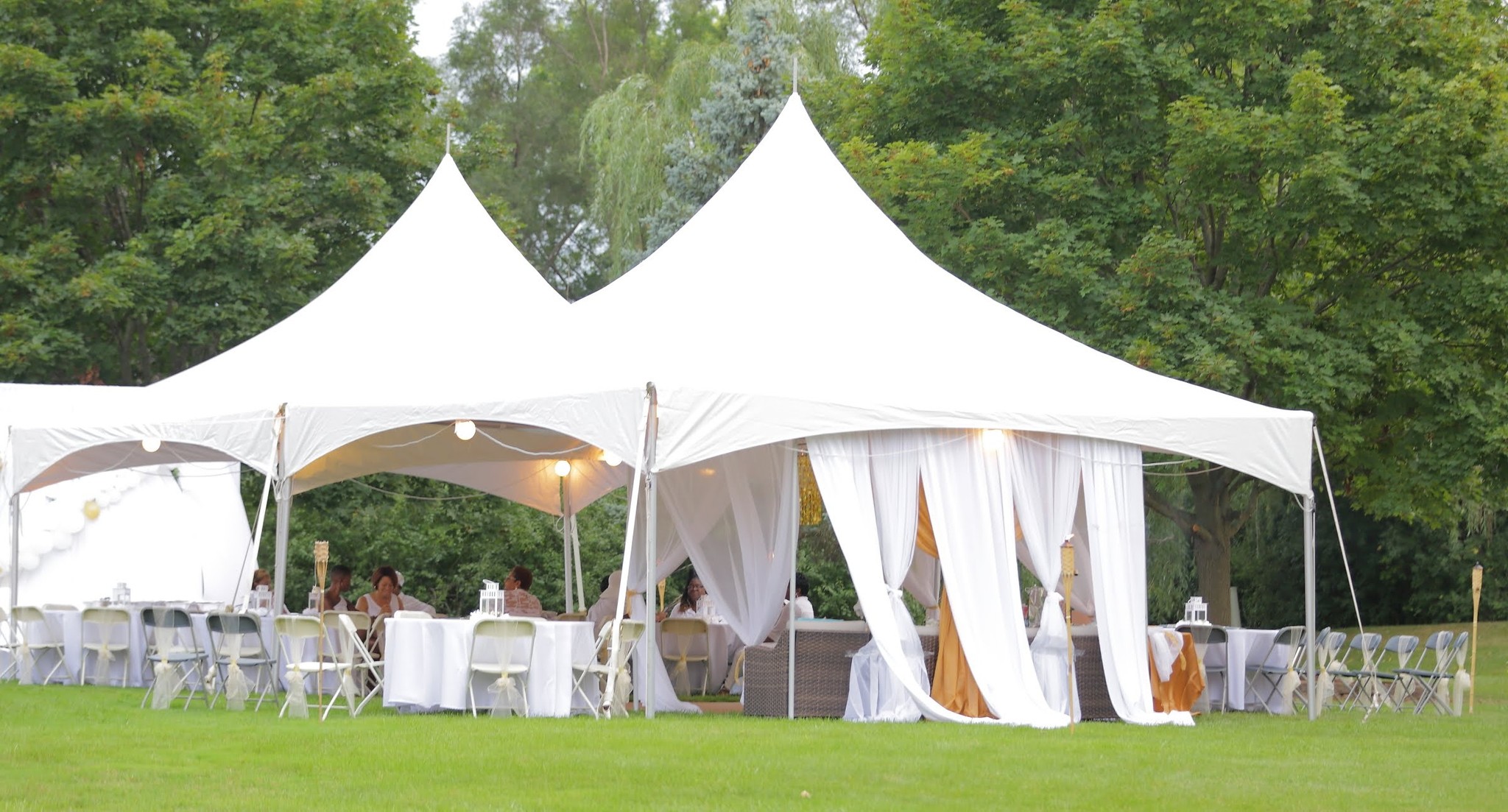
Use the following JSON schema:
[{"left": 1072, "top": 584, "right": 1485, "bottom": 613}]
[
  {"left": 599, "top": 570, "right": 621, "bottom": 598},
  {"left": 395, "top": 570, "right": 405, "bottom": 587}
]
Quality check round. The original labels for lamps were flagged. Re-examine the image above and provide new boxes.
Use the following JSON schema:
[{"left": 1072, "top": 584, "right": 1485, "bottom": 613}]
[
  {"left": 453, "top": 419, "right": 476, "bottom": 441},
  {"left": 556, "top": 461, "right": 573, "bottom": 482},
  {"left": 139, "top": 439, "right": 160, "bottom": 457}
]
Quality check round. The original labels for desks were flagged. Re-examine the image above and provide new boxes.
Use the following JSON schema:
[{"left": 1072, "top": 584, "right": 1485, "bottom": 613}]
[
  {"left": 1150, "top": 624, "right": 1304, "bottom": 718},
  {"left": 382, "top": 616, "right": 599, "bottom": 718},
  {"left": 654, "top": 617, "right": 738, "bottom": 696}
]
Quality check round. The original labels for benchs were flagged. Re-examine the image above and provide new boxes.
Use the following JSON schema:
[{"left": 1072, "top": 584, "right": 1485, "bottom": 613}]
[{"left": 746, "top": 617, "right": 1120, "bottom": 719}]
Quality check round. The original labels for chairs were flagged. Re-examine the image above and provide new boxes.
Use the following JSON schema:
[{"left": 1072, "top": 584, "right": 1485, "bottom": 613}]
[
  {"left": 1, "top": 599, "right": 713, "bottom": 720},
  {"left": 1173, "top": 626, "right": 1467, "bottom": 719}
]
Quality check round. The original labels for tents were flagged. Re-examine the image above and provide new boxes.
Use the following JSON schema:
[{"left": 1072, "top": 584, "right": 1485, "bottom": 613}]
[
  {"left": 0, "top": 382, "right": 260, "bottom": 653},
  {"left": 8, "top": 57, "right": 1317, "bottom": 729}
]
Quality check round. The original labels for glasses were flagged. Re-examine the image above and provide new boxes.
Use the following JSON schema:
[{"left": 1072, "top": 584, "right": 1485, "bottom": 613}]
[
  {"left": 687, "top": 584, "right": 702, "bottom": 590},
  {"left": 505, "top": 575, "right": 517, "bottom": 581}
]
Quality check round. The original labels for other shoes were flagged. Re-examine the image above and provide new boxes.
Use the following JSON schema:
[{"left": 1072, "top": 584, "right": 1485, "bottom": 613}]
[{"left": 718, "top": 687, "right": 728, "bottom": 695}]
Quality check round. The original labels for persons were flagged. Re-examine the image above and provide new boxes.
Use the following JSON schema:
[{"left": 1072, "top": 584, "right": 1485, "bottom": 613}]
[{"left": 248, "top": 564, "right": 814, "bottom": 696}]
